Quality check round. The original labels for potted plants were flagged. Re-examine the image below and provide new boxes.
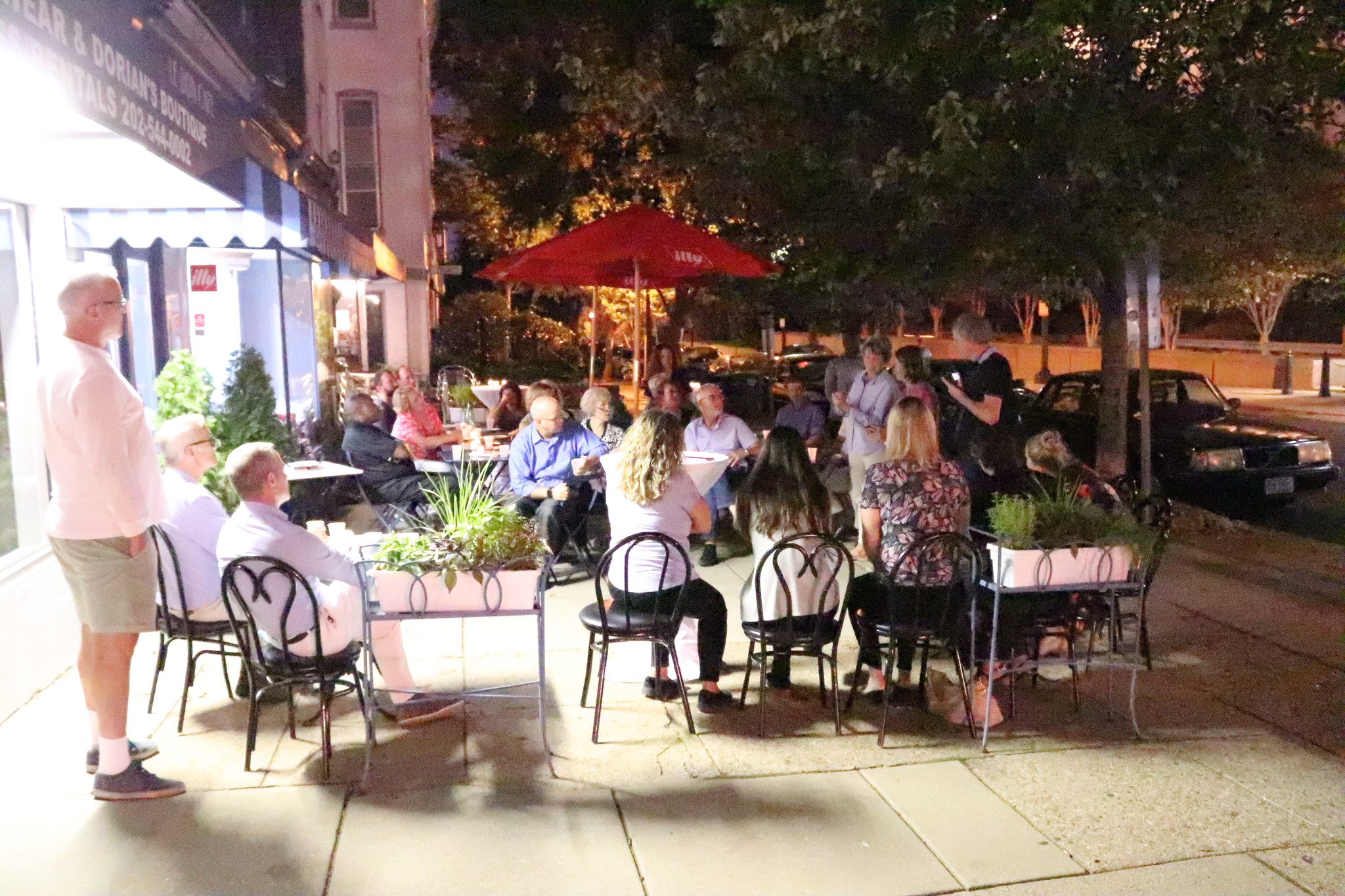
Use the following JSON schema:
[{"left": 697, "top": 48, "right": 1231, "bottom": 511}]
[
  {"left": 352, "top": 451, "right": 549, "bottom": 623},
  {"left": 976, "top": 469, "right": 1156, "bottom": 594}
]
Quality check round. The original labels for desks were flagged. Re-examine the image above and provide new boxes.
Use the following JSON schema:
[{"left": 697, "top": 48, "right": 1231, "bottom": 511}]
[
  {"left": 438, "top": 414, "right": 520, "bottom": 493},
  {"left": 803, "top": 439, "right": 829, "bottom": 478},
  {"left": 670, "top": 447, "right": 741, "bottom": 504},
  {"left": 274, "top": 447, "right": 370, "bottom": 538}
]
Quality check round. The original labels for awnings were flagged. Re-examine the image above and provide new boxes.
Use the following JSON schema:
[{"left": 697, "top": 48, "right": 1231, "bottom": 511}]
[{"left": 0, "top": 0, "right": 411, "bottom": 287}]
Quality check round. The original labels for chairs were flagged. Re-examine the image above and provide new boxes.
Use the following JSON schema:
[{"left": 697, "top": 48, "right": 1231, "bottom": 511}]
[
  {"left": 852, "top": 528, "right": 979, "bottom": 755},
  {"left": 137, "top": 515, "right": 248, "bottom": 738},
  {"left": 217, "top": 552, "right": 368, "bottom": 785},
  {"left": 576, "top": 527, "right": 699, "bottom": 748},
  {"left": 999, "top": 470, "right": 1186, "bottom": 716},
  {"left": 732, "top": 528, "right": 857, "bottom": 745}
]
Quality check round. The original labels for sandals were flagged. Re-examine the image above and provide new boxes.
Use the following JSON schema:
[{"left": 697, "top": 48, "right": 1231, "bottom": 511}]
[
  {"left": 1001, "top": 654, "right": 1036, "bottom": 686},
  {"left": 975, "top": 657, "right": 1007, "bottom": 682}
]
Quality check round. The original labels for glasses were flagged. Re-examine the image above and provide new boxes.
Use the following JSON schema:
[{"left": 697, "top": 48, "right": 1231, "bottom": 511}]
[
  {"left": 536, "top": 413, "right": 563, "bottom": 424},
  {"left": 597, "top": 406, "right": 615, "bottom": 413},
  {"left": 185, "top": 436, "right": 216, "bottom": 456},
  {"left": 86, "top": 297, "right": 127, "bottom": 315},
  {"left": 697, "top": 392, "right": 726, "bottom": 401}
]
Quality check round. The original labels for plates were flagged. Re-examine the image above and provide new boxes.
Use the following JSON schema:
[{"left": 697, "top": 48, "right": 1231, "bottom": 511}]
[
  {"left": 482, "top": 428, "right": 500, "bottom": 430},
  {"left": 495, "top": 435, "right": 508, "bottom": 438},
  {"left": 442, "top": 424, "right": 456, "bottom": 427}
]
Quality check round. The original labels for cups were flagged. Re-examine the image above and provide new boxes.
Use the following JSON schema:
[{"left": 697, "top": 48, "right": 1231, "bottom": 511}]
[
  {"left": 306, "top": 520, "right": 324, "bottom": 535},
  {"left": 327, "top": 522, "right": 346, "bottom": 536},
  {"left": 484, "top": 435, "right": 494, "bottom": 448},
  {"left": 451, "top": 445, "right": 462, "bottom": 460},
  {"left": 571, "top": 458, "right": 585, "bottom": 475},
  {"left": 486, "top": 419, "right": 494, "bottom": 429},
  {"left": 498, "top": 445, "right": 509, "bottom": 458}
]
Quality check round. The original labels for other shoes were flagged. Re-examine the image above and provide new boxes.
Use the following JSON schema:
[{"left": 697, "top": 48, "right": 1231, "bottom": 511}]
[
  {"left": 858, "top": 684, "right": 884, "bottom": 703},
  {"left": 696, "top": 689, "right": 732, "bottom": 714},
  {"left": 642, "top": 674, "right": 682, "bottom": 699},
  {"left": 394, "top": 691, "right": 481, "bottom": 727},
  {"left": 890, "top": 683, "right": 916, "bottom": 700},
  {"left": 767, "top": 672, "right": 790, "bottom": 689},
  {"left": 235, "top": 669, "right": 288, "bottom": 703}
]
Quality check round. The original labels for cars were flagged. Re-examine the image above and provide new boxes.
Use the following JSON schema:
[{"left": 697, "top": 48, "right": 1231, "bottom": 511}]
[
  {"left": 886, "top": 358, "right": 1040, "bottom": 459},
  {"left": 1029, "top": 366, "right": 1341, "bottom": 520},
  {"left": 680, "top": 343, "right": 780, "bottom": 383},
  {"left": 770, "top": 343, "right": 838, "bottom": 389}
]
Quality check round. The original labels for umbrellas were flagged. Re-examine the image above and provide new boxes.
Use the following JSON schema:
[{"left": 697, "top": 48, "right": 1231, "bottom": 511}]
[{"left": 473, "top": 194, "right": 786, "bottom": 427}]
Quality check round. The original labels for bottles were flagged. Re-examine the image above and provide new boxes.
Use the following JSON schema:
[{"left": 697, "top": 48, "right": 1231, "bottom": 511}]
[{"left": 465, "top": 401, "right": 476, "bottom": 428}]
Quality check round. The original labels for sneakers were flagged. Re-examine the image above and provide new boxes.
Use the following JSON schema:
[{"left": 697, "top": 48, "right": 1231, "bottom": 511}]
[
  {"left": 91, "top": 761, "right": 186, "bottom": 800},
  {"left": 1038, "top": 656, "right": 1087, "bottom": 679},
  {"left": 86, "top": 736, "right": 159, "bottom": 773},
  {"left": 698, "top": 544, "right": 719, "bottom": 566},
  {"left": 713, "top": 507, "right": 753, "bottom": 556}
]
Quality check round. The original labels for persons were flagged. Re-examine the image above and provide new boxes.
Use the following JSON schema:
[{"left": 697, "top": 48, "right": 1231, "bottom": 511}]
[
  {"left": 599, "top": 409, "right": 742, "bottom": 713},
  {"left": 215, "top": 442, "right": 464, "bottom": 727},
  {"left": 845, "top": 397, "right": 1002, "bottom": 726},
  {"left": 42, "top": 270, "right": 184, "bottom": 798},
  {"left": 344, "top": 312, "right": 1141, "bottom": 656},
  {"left": 736, "top": 425, "right": 840, "bottom": 686},
  {"left": 148, "top": 413, "right": 296, "bottom": 705}
]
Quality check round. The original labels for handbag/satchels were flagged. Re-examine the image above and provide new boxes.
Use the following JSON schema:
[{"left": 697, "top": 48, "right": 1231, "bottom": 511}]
[{"left": 924, "top": 665, "right": 1004, "bottom": 727}]
